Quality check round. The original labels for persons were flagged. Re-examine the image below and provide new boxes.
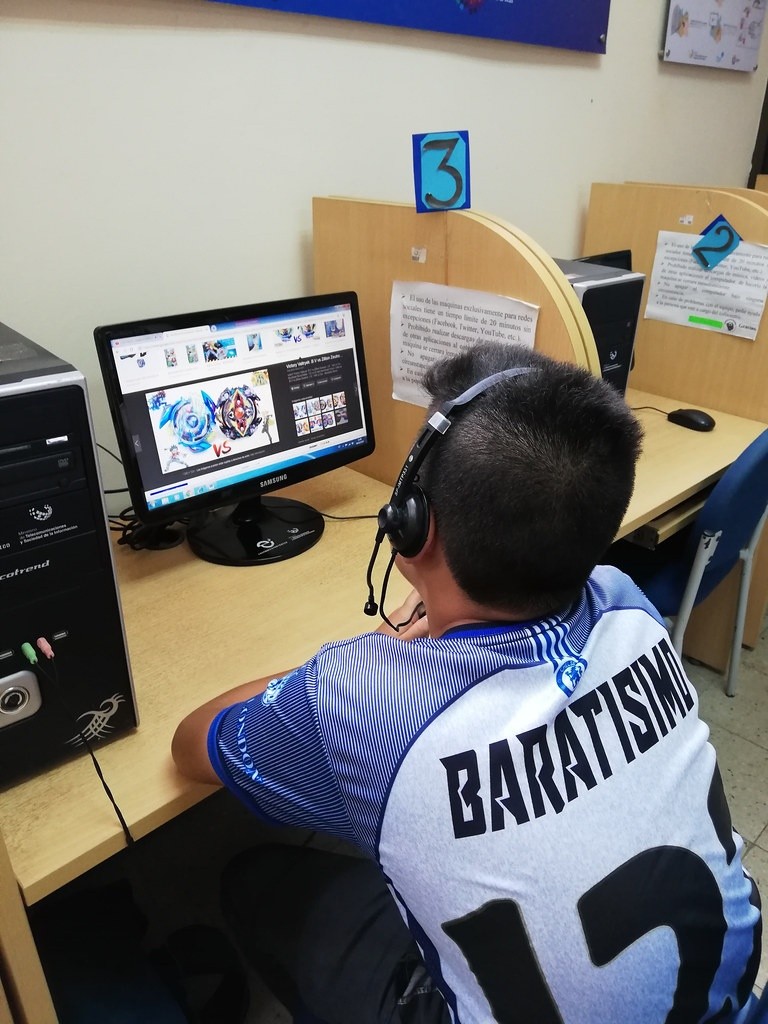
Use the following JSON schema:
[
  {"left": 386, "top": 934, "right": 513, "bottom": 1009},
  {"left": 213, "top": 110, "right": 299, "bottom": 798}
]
[{"left": 171, "top": 340, "right": 768, "bottom": 1024}]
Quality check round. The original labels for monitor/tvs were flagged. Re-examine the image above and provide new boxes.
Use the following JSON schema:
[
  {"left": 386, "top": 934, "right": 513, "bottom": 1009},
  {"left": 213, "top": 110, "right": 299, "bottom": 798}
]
[
  {"left": 95, "top": 291, "right": 376, "bottom": 566},
  {"left": 570, "top": 250, "right": 636, "bottom": 371}
]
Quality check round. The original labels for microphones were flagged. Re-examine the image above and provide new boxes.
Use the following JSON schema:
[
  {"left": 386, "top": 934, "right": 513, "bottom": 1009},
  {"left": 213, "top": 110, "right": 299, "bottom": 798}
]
[{"left": 365, "top": 527, "right": 386, "bottom": 616}]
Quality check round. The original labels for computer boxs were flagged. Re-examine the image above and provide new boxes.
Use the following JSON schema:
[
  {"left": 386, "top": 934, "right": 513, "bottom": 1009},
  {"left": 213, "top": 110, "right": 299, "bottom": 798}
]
[
  {"left": 0, "top": 322, "right": 141, "bottom": 791},
  {"left": 549, "top": 258, "right": 646, "bottom": 400}
]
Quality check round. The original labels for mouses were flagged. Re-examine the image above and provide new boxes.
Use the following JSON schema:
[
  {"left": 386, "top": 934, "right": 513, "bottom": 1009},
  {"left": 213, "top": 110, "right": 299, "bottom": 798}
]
[{"left": 667, "top": 409, "right": 716, "bottom": 433}]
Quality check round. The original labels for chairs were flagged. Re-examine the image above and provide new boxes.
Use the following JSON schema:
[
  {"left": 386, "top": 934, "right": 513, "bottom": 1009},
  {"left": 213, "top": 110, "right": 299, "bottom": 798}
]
[{"left": 597, "top": 427, "right": 768, "bottom": 698}]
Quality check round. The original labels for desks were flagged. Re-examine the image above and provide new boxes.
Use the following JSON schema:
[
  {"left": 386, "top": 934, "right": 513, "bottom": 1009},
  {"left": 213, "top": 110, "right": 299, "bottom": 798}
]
[
  {"left": 0, "top": 464, "right": 415, "bottom": 911},
  {"left": 612, "top": 386, "right": 768, "bottom": 544}
]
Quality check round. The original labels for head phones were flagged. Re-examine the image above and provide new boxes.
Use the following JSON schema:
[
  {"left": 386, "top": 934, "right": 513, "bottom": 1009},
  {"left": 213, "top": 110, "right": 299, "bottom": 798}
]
[{"left": 377, "top": 363, "right": 546, "bottom": 557}]
